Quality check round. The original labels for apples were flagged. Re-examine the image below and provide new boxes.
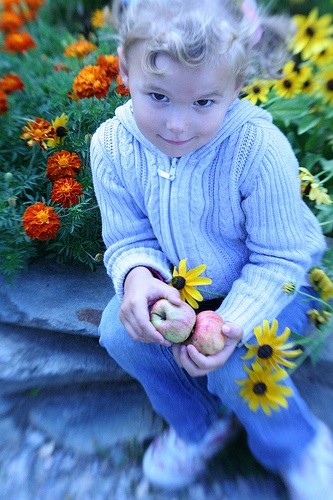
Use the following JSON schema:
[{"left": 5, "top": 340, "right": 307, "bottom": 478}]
[{"left": 152, "top": 299, "right": 226, "bottom": 356}]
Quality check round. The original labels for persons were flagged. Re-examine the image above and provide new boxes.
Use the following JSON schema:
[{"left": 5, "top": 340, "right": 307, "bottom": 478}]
[{"left": 90, "top": 0, "right": 332, "bottom": 500}]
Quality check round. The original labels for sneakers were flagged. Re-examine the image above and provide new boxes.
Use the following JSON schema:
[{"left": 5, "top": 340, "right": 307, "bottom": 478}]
[
  {"left": 142, "top": 415, "right": 231, "bottom": 489},
  {"left": 285, "top": 420, "right": 333, "bottom": 500}
]
